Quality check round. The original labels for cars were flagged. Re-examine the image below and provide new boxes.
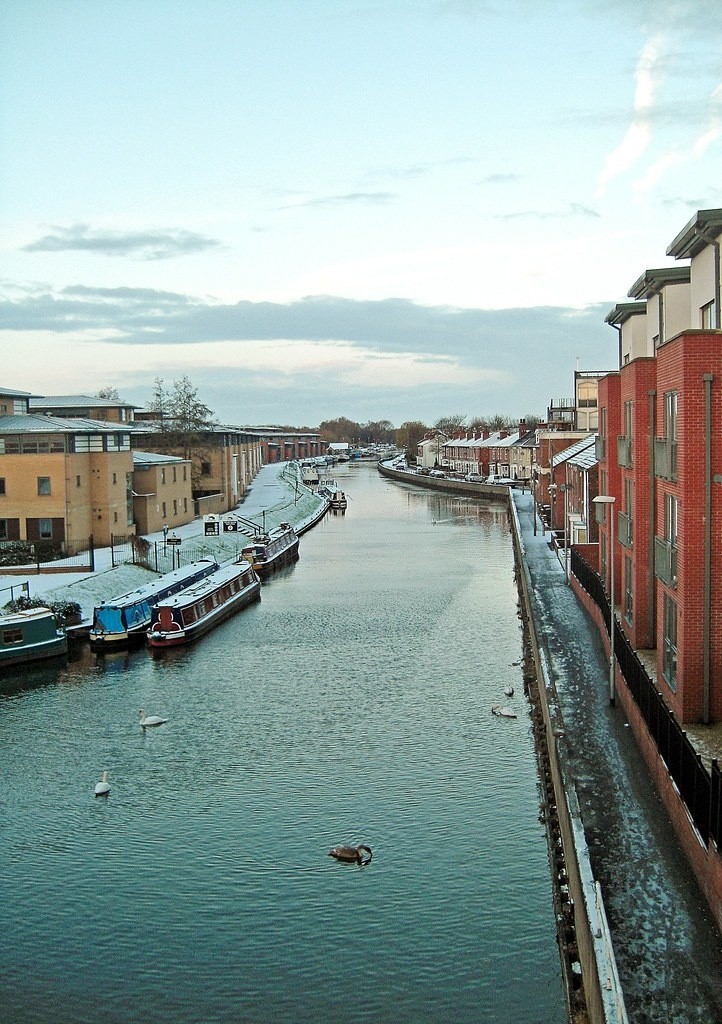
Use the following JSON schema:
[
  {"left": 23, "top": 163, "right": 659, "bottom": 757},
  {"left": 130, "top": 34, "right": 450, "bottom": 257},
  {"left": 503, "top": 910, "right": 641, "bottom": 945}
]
[{"left": 392, "top": 454, "right": 519, "bottom": 489}]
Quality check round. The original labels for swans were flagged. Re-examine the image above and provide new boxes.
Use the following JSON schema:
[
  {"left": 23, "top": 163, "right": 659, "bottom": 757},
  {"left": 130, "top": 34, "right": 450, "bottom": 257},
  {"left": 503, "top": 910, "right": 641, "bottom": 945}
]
[
  {"left": 504, "top": 683, "right": 514, "bottom": 697},
  {"left": 431, "top": 518, "right": 436, "bottom": 524},
  {"left": 491, "top": 704, "right": 518, "bottom": 718},
  {"left": 94, "top": 770, "right": 111, "bottom": 795},
  {"left": 138, "top": 709, "right": 171, "bottom": 725},
  {"left": 329, "top": 844, "right": 373, "bottom": 859}
]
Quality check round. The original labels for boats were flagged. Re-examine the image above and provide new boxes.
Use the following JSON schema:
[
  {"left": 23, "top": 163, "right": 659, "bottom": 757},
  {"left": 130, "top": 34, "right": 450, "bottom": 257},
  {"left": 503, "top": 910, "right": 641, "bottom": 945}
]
[
  {"left": 239, "top": 522, "right": 299, "bottom": 576},
  {"left": 146, "top": 561, "right": 261, "bottom": 647},
  {"left": 299, "top": 447, "right": 385, "bottom": 509},
  {"left": 89, "top": 556, "right": 221, "bottom": 644},
  {"left": 0, "top": 607, "right": 68, "bottom": 667}
]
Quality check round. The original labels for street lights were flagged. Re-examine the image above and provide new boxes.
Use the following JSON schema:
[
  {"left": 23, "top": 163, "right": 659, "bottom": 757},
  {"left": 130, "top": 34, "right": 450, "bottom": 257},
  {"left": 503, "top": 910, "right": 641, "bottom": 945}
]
[{"left": 592, "top": 496, "right": 616, "bottom": 708}]
